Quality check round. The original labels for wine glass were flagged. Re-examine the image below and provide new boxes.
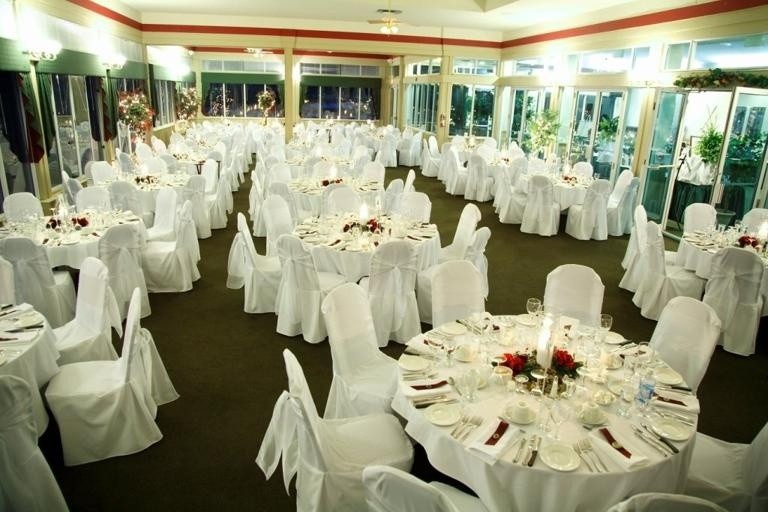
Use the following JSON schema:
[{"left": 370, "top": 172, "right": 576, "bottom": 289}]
[
  {"left": 443, "top": 295, "right": 655, "bottom": 441},
  {"left": 638, "top": 342, "right": 651, "bottom": 368},
  {"left": 2, "top": 206, "right": 140, "bottom": 246},
  {"left": 103, "top": 168, "right": 192, "bottom": 190}
]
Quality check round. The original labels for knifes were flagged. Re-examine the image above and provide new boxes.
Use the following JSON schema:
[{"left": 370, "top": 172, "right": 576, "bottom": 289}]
[
  {"left": 630, "top": 421, "right": 679, "bottom": 458},
  {"left": 513, "top": 434, "right": 542, "bottom": 468}
]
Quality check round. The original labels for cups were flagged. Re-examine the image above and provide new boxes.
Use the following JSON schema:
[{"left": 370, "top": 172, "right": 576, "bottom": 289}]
[{"left": 600, "top": 314, "right": 613, "bottom": 332}]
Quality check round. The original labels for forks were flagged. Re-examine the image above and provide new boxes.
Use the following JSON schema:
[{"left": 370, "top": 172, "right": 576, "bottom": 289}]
[
  {"left": 655, "top": 384, "right": 693, "bottom": 395},
  {"left": 450, "top": 415, "right": 483, "bottom": 441},
  {"left": 573, "top": 439, "right": 610, "bottom": 473}
]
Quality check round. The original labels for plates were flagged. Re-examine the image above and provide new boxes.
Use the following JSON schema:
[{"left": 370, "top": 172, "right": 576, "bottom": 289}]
[
  {"left": 651, "top": 418, "right": 691, "bottom": 442},
  {"left": 424, "top": 404, "right": 460, "bottom": 426},
  {"left": 296, "top": 211, "right": 437, "bottom": 252},
  {"left": 516, "top": 317, "right": 538, "bottom": 326},
  {"left": 437, "top": 322, "right": 468, "bottom": 335},
  {"left": 12, "top": 316, "right": 43, "bottom": 327},
  {"left": 652, "top": 369, "right": 684, "bottom": 384},
  {"left": 539, "top": 444, "right": 581, "bottom": 472},
  {"left": 602, "top": 332, "right": 624, "bottom": 343},
  {"left": 399, "top": 355, "right": 430, "bottom": 371},
  {"left": 683, "top": 219, "right": 763, "bottom": 255}
]
